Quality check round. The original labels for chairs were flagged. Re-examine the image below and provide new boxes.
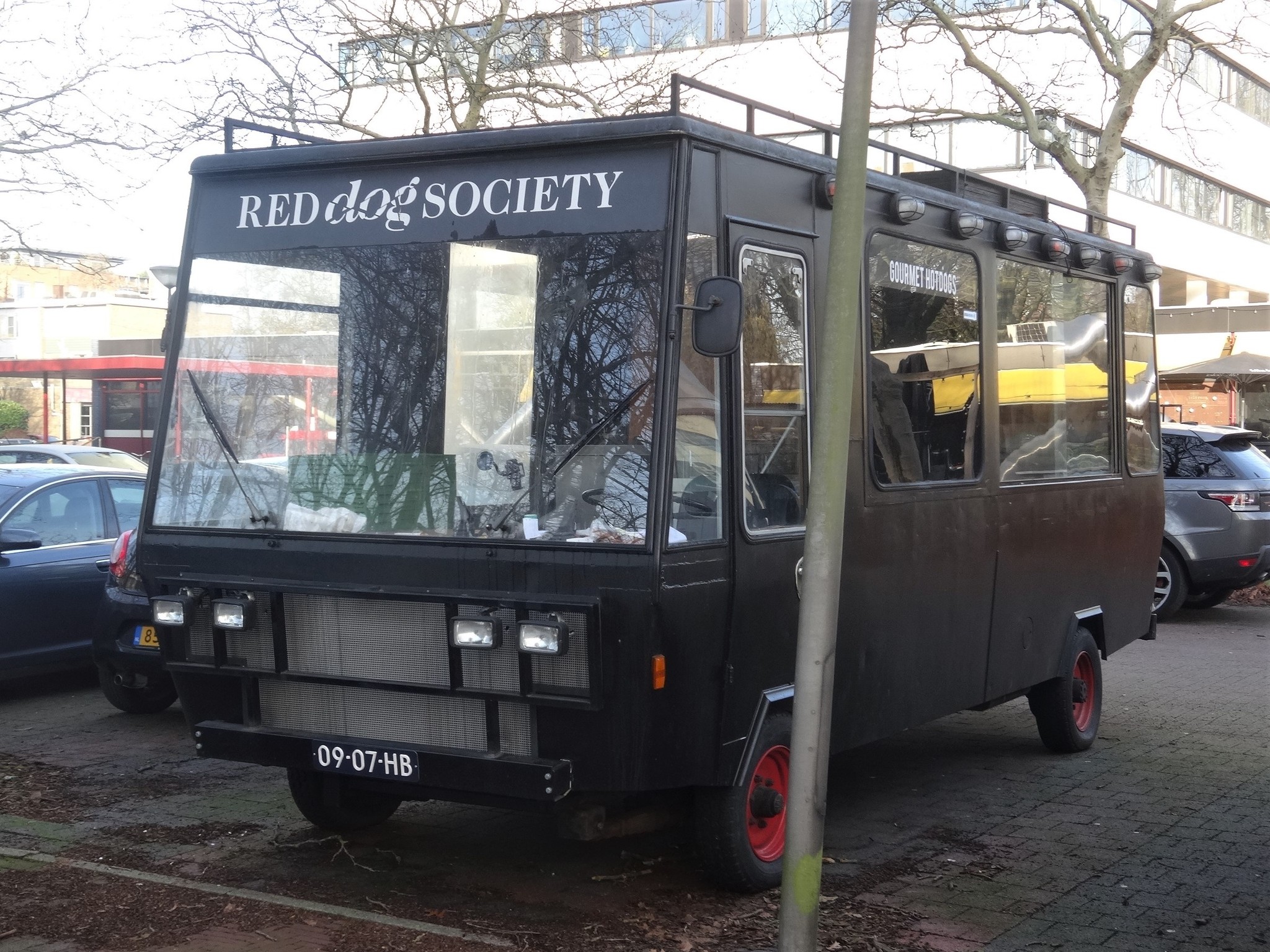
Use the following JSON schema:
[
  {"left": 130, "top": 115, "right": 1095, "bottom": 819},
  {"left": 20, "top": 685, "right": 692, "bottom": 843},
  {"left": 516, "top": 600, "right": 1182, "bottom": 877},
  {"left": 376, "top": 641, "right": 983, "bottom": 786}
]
[{"left": 57, "top": 496, "right": 89, "bottom": 543}]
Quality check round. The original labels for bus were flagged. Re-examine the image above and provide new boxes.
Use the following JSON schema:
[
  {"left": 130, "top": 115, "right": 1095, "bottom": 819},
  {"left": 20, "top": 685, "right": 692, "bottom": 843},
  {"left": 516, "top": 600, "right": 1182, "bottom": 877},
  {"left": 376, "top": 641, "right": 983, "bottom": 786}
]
[{"left": 102, "top": 76, "right": 1163, "bottom": 888}]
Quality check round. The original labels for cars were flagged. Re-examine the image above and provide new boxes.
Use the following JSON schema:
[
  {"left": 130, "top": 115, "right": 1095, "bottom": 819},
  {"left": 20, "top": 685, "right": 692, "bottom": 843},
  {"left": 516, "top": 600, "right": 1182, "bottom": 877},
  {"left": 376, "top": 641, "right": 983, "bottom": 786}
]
[{"left": 0, "top": 438, "right": 296, "bottom": 694}]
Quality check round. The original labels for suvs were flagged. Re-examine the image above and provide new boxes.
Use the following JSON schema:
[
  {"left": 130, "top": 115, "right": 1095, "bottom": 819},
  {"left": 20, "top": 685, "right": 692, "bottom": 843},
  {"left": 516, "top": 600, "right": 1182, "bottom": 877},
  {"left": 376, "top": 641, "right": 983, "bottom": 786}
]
[{"left": 1123, "top": 413, "right": 1270, "bottom": 625}]
